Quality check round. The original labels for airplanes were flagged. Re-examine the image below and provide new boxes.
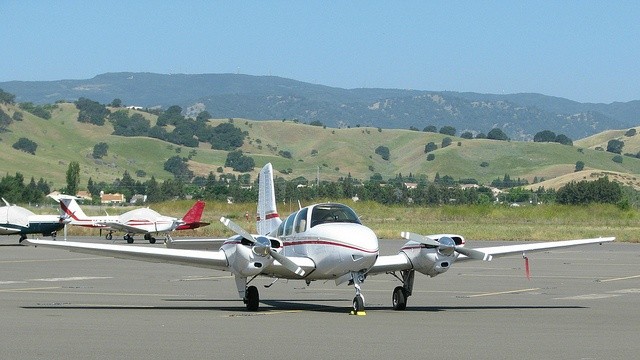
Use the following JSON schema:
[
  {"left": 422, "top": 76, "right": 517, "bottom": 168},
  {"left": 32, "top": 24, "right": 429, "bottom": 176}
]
[
  {"left": 21, "top": 162, "right": 616, "bottom": 314},
  {"left": 0, "top": 197, "right": 75, "bottom": 243},
  {"left": 45, "top": 191, "right": 210, "bottom": 244}
]
